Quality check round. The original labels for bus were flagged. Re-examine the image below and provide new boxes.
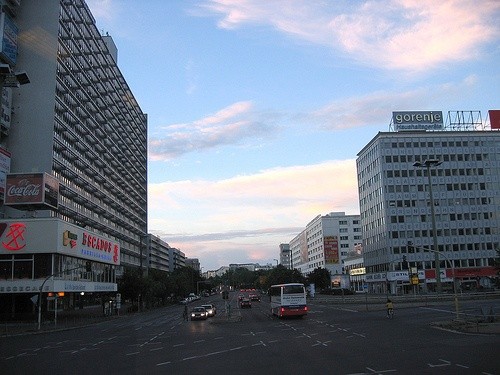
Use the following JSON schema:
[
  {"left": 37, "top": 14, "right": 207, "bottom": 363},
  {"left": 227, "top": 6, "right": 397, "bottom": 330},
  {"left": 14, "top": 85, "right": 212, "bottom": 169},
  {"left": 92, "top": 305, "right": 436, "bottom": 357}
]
[{"left": 268, "top": 283, "right": 307, "bottom": 319}]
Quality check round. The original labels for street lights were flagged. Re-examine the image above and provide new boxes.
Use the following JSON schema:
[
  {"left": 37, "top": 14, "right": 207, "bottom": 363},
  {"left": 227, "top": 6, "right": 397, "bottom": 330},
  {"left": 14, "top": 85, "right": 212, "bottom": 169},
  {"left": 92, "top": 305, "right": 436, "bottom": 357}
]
[
  {"left": 274, "top": 259, "right": 278, "bottom": 268},
  {"left": 412, "top": 159, "right": 444, "bottom": 295},
  {"left": 285, "top": 249, "right": 292, "bottom": 270},
  {"left": 137, "top": 234, "right": 151, "bottom": 312}
]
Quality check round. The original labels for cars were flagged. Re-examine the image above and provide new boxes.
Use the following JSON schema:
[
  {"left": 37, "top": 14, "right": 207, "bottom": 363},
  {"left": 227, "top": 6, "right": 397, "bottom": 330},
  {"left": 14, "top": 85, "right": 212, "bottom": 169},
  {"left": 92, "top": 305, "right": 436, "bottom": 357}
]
[
  {"left": 238, "top": 289, "right": 260, "bottom": 309},
  {"left": 201, "top": 304, "right": 216, "bottom": 317},
  {"left": 191, "top": 306, "right": 208, "bottom": 320},
  {"left": 178, "top": 291, "right": 217, "bottom": 305}
]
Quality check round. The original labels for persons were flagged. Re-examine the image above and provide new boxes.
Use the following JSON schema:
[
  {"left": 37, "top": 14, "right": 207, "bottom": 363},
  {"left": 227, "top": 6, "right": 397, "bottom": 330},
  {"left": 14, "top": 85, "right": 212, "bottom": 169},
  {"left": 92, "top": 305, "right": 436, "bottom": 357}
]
[
  {"left": 385, "top": 299, "right": 393, "bottom": 317},
  {"left": 183, "top": 306, "right": 189, "bottom": 320}
]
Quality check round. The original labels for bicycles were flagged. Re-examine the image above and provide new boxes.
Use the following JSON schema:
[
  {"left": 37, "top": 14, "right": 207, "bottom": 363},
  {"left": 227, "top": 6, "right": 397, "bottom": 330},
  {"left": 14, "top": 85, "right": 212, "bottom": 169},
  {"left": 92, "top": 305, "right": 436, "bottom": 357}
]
[
  {"left": 385, "top": 308, "right": 393, "bottom": 320},
  {"left": 183, "top": 311, "right": 189, "bottom": 321}
]
